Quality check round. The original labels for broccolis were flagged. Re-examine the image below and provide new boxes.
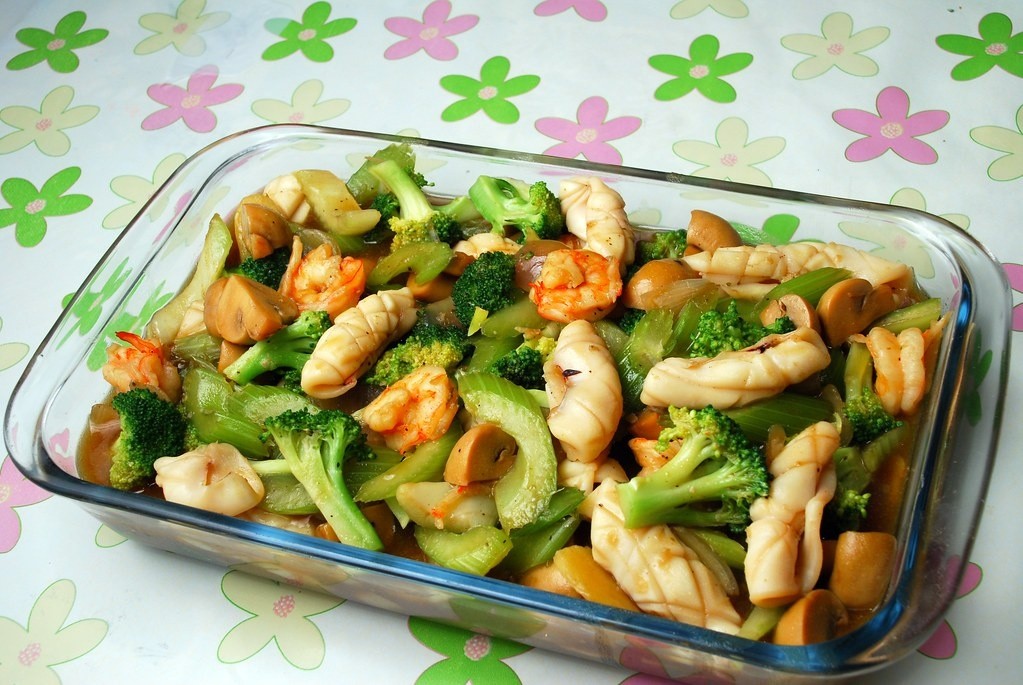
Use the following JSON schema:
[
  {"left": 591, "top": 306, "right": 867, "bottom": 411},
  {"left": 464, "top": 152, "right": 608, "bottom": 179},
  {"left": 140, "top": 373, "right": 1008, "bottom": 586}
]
[{"left": 109, "top": 146, "right": 945, "bottom": 643}]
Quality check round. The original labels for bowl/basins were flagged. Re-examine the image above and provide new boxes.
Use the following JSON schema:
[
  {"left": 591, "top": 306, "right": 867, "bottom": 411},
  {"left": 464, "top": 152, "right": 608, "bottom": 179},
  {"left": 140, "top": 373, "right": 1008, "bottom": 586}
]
[{"left": 4, "top": 123, "right": 1012, "bottom": 685}]
